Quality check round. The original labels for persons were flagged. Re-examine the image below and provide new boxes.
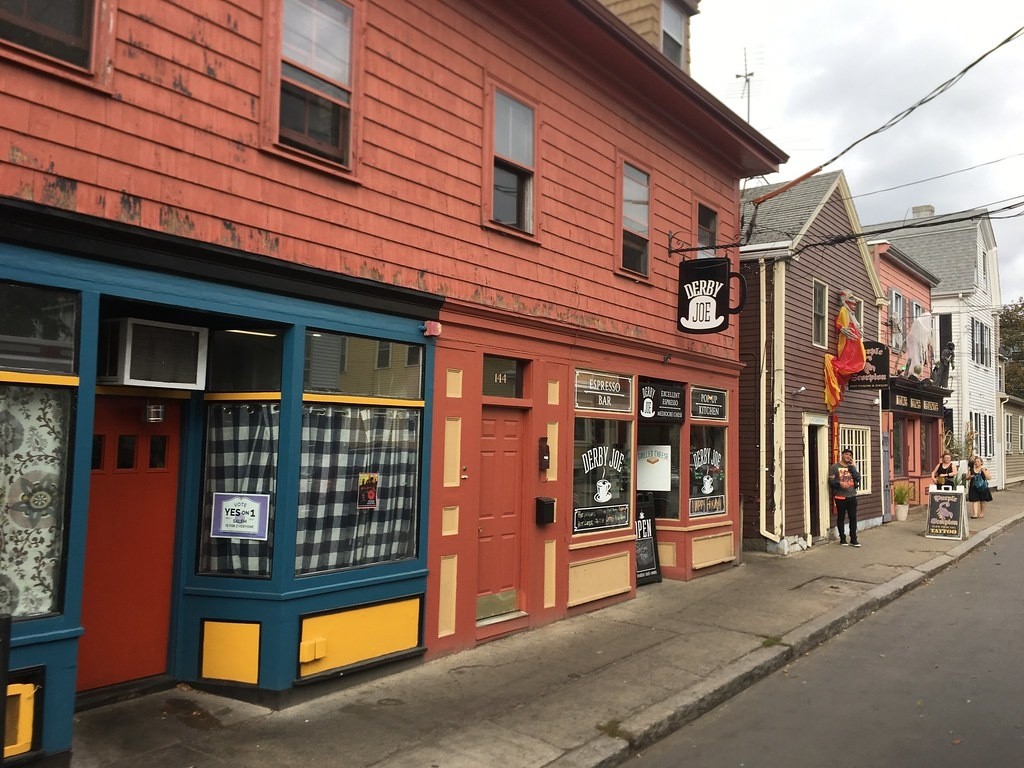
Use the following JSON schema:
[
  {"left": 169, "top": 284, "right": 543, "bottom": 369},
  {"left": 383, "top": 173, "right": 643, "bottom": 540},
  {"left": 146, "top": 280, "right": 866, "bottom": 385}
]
[
  {"left": 930, "top": 452, "right": 958, "bottom": 486},
  {"left": 935, "top": 341, "right": 955, "bottom": 387},
  {"left": 828, "top": 449, "right": 862, "bottom": 548},
  {"left": 965, "top": 456, "right": 993, "bottom": 518}
]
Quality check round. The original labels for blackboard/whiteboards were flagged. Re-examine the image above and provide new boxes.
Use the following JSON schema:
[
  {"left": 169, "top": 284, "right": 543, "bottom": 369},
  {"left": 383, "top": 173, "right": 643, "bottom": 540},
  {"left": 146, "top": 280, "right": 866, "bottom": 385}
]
[
  {"left": 926, "top": 492, "right": 963, "bottom": 539},
  {"left": 635, "top": 492, "right": 663, "bottom": 588}
]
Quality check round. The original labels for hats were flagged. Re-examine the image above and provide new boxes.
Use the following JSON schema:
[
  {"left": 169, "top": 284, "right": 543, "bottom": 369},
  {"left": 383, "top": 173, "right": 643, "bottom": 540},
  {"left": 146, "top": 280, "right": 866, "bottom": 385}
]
[{"left": 842, "top": 449, "right": 854, "bottom": 457}]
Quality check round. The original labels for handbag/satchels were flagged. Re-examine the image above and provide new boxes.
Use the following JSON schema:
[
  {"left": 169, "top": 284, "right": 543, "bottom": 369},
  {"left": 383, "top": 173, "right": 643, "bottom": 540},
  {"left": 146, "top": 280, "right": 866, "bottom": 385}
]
[
  {"left": 848, "top": 465, "right": 858, "bottom": 489},
  {"left": 936, "top": 476, "right": 945, "bottom": 484},
  {"left": 979, "top": 468, "right": 988, "bottom": 489}
]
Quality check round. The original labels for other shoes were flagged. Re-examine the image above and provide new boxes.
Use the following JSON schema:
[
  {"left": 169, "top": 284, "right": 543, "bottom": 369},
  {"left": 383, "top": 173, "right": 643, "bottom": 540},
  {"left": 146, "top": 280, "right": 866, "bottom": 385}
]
[
  {"left": 849, "top": 541, "right": 861, "bottom": 547},
  {"left": 971, "top": 516, "right": 978, "bottom": 519},
  {"left": 979, "top": 512, "right": 985, "bottom": 518},
  {"left": 840, "top": 540, "right": 849, "bottom": 546}
]
